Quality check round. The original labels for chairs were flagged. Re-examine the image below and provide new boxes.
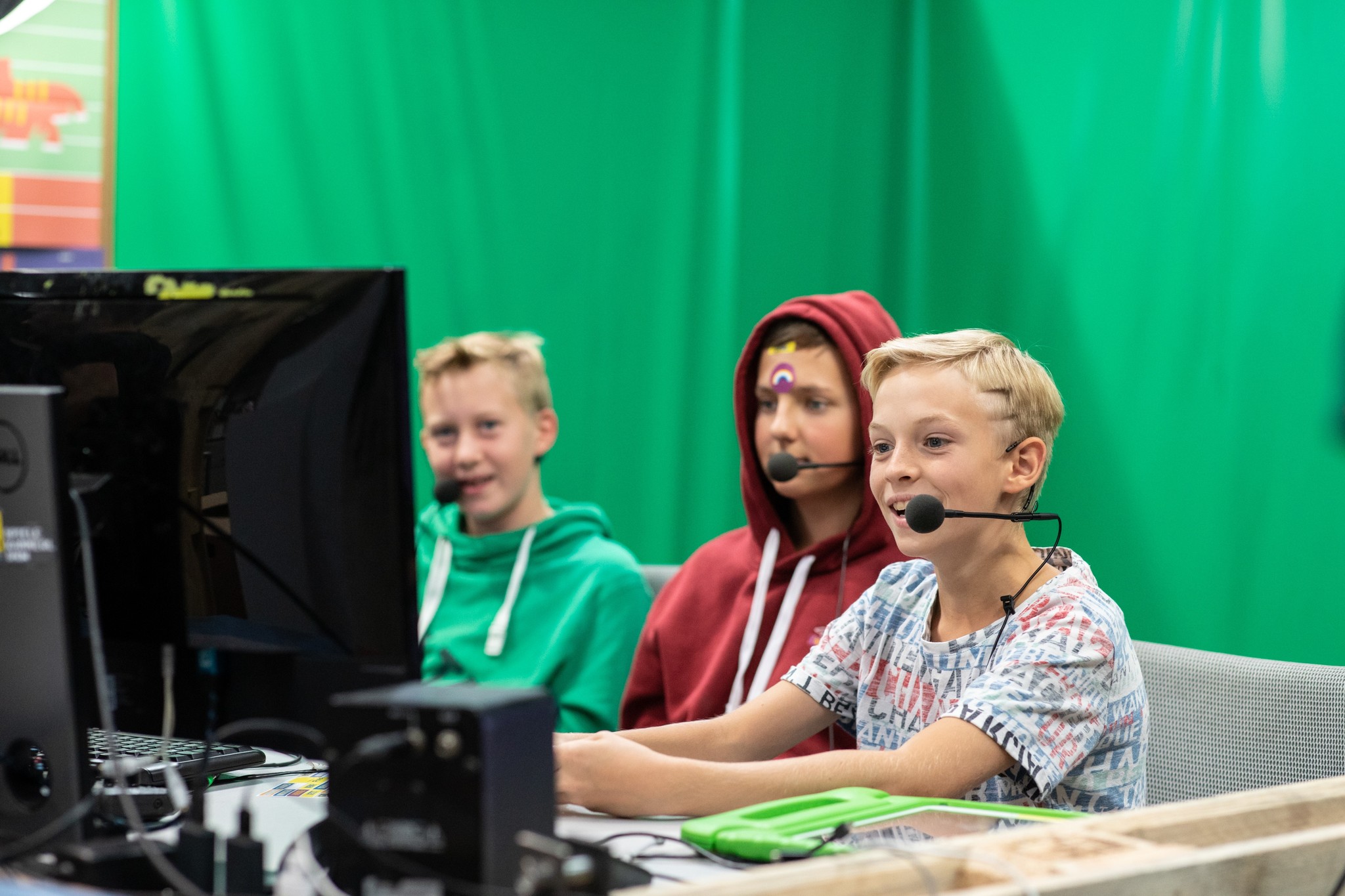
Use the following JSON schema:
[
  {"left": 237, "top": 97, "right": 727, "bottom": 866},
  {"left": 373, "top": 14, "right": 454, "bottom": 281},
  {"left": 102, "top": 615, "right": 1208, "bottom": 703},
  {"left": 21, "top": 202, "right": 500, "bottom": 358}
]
[{"left": 1137, "top": 640, "right": 1345, "bottom": 805}]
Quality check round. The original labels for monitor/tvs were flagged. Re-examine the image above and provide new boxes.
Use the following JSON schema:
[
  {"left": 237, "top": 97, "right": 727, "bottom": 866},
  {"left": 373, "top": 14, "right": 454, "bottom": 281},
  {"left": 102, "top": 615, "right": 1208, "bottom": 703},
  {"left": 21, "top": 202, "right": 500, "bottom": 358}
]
[{"left": 0, "top": 268, "right": 422, "bottom": 850}]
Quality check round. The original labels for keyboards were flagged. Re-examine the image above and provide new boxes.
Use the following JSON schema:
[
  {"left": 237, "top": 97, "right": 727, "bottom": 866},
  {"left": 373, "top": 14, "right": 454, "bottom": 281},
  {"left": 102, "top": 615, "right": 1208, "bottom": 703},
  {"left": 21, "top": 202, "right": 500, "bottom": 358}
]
[{"left": 84, "top": 728, "right": 265, "bottom": 788}]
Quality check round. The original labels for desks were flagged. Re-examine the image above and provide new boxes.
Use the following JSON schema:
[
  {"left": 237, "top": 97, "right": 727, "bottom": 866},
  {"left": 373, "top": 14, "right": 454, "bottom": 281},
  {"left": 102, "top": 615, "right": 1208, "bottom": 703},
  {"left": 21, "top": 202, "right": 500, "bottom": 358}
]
[{"left": 0, "top": 749, "right": 741, "bottom": 896}]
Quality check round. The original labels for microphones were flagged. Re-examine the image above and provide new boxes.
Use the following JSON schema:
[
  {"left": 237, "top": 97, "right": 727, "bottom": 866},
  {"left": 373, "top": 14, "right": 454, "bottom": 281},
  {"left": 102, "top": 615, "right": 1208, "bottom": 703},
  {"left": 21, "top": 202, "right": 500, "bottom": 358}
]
[
  {"left": 434, "top": 479, "right": 459, "bottom": 506},
  {"left": 767, "top": 452, "right": 863, "bottom": 482},
  {"left": 904, "top": 494, "right": 1033, "bottom": 534}
]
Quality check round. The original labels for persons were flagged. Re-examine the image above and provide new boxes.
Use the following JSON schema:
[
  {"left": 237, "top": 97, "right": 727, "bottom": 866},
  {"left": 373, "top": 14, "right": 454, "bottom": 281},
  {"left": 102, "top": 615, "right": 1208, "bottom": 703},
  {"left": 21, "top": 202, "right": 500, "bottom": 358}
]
[
  {"left": 553, "top": 329, "right": 1149, "bottom": 823},
  {"left": 620, "top": 290, "right": 928, "bottom": 768},
  {"left": 414, "top": 333, "right": 654, "bottom": 733}
]
